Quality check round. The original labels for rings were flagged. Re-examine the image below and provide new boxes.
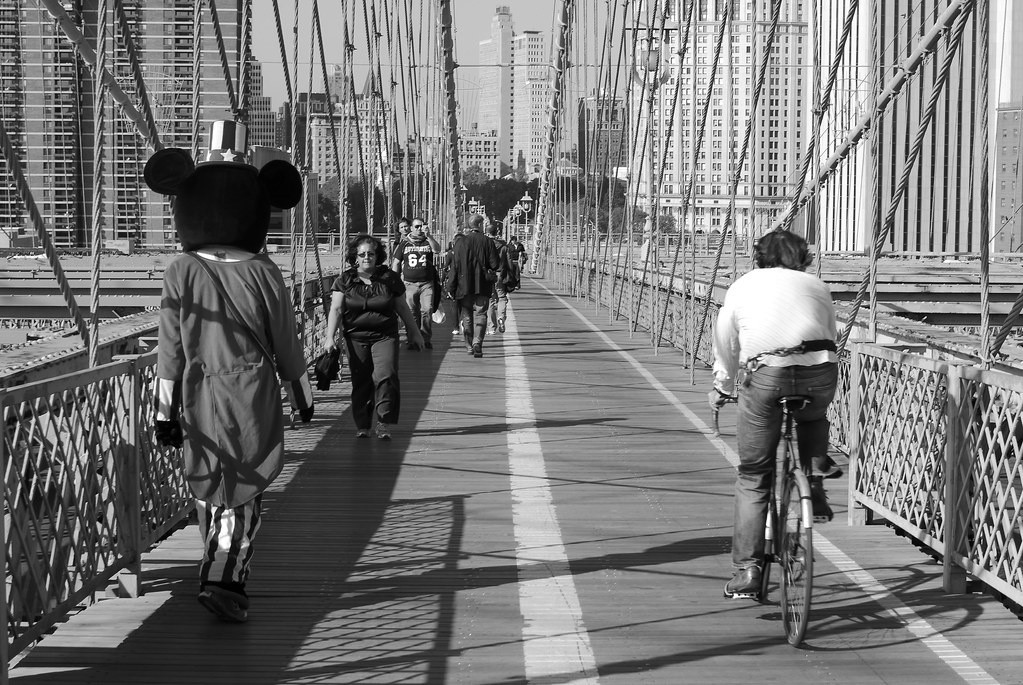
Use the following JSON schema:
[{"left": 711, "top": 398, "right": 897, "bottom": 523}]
[{"left": 712, "top": 403, "right": 714, "bottom": 404}]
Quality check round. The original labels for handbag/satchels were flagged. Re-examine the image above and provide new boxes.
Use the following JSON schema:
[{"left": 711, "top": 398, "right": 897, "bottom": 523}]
[
  {"left": 484, "top": 268, "right": 498, "bottom": 283},
  {"left": 431, "top": 301, "right": 446, "bottom": 324}
]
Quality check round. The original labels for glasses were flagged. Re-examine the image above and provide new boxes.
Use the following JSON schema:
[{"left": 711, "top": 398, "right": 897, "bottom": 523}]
[
  {"left": 414, "top": 225, "right": 422, "bottom": 228},
  {"left": 357, "top": 253, "right": 377, "bottom": 257}
]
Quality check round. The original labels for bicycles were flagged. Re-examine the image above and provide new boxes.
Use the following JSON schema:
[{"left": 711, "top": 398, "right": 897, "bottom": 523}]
[{"left": 712, "top": 393, "right": 830, "bottom": 646}]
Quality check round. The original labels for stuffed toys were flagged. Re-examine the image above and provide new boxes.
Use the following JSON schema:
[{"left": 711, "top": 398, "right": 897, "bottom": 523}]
[{"left": 143, "top": 148, "right": 315, "bottom": 619}]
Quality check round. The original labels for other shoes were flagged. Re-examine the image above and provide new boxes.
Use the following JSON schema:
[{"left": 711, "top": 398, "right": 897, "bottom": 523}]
[
  {"left": 408, "top": 344, "right": 416, "bottom": 349},
  {"left": 810, "top": 482, "right": 834, "bottom": 522},
  {"left": 425, "top": 342, "right": 432, "bottom": 348},
  {"left": 498, "top": 317, "right": 505, "bottom": 333},
  {"left": 452, "top": 329, "right": 460, "bottom": 335},
  {"left": 724, "top": 565, "right": 760, "bottom": 597},
  {"left": 473, "top": 343, "right": 483, "bottom": 357},
  {"left": 467, "top": 347, "right": 474, "bottom": 354},
  {"left": 485, "top": 327, "right": 497, "bottom": 334}
]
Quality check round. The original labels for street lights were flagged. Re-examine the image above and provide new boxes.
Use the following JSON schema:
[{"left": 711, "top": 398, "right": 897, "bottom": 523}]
[{"left": 579, "top": 215, "right": 593, "bottom": 246}]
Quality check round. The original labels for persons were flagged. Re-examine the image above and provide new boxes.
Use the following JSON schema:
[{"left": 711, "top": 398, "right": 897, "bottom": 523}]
[
  {"left": 393, "top": 217, "right": 440, "bottom": 348},
  {"left": 486, "top": 224, "right": 512, "bottom": 334},
  {"left": 324, "top": 234, "right": 426, "bottom": 441},
  {"left": 393, "top": 218, "right": 412, "bottom": 273},
  {"left": 446, "top": 214, "right": 499, "bottom": 356},
  {"left": 707, "top": 228, "right": 840, "bottom": 594},
  {"left": 509, "top": 236, "right": 525, "bottom": 289}
]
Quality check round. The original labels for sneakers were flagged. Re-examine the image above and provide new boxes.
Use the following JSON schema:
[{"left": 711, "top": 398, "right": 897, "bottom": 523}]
[
  {"left": 374, "top": 421, "right": 392, "bottom": 439},
  {"left": 356, "top": 428, "right": 370, "bottom": 437}
]
